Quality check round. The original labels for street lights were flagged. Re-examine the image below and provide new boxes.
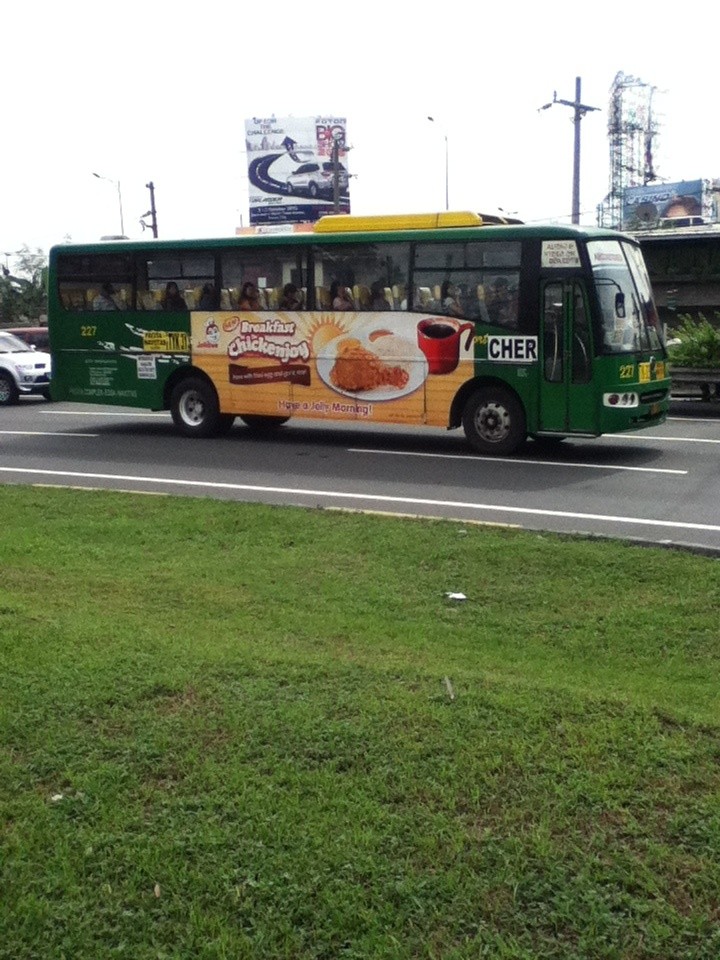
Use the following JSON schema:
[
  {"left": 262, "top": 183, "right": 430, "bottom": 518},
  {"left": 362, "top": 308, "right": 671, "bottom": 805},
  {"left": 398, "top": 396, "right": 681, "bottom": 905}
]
[
  {"left": 93, "top": 173, "right": 125, "bottom": 235},
  {"left": 427, "top": 115, "right": 451, "bottom": 214}
]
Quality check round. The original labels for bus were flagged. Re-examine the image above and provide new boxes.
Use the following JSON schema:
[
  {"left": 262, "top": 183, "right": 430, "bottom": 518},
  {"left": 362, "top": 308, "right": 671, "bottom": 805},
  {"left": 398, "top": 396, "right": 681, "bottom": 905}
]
[{"left": 48, "top": 210, "right": 673, "bottom": 455}]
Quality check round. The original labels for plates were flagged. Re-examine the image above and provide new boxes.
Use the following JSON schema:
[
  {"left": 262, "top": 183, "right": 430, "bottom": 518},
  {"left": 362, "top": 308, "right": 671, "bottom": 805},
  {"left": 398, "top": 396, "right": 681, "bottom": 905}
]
[{"left": 316, "top": 328, "right": 430, "bottom": 402}]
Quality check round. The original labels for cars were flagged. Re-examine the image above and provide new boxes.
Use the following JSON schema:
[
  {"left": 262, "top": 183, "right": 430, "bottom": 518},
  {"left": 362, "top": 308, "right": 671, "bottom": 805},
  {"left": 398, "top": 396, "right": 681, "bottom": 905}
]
[
  {"left": 0, "top": 332, "right": 51, "bottom": 405},
  {"left": 2, "top": 327, "right": 47, "bottom": 353},
  {"left": 284, "top": 160, "right": 348, "bottom": 196}
]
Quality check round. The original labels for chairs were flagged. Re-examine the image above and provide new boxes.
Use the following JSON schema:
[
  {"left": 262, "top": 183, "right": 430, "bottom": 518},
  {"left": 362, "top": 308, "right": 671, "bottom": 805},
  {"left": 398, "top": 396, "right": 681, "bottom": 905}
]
[
  {"left": 432, "top": 285, "right": 442, "bottom": 312},
  {"left": 194, "top": 286, "right": 203, "bottom": 310},
  {"left": 86, "top": 287, "right": 103, "bottom": 310},
  {"left": 417, "top": 286, "right": 437, "bottom": 312},
  {"left": 184, "top": 290, "right": 195, "bottom": 309},
  {"left": 220, "top": 287, "right": 307, "bottom": 310},
  {"left": 155, "top": 290, "right": 166, "bottom": 311},
  {"left": 392, "top": 284, "right": 406, "bottom": 310},
  {"left": 384, "top": 287, "right": 394, "bottom": 310},
  {"left": 121, "top": 288, "right": 140, "bottom": 310},
  {"left": 315, "top": 286, "right": 333, "bottom": 310},
  {"left": 353, "top": 284, "right": 374, "bottom": 311},
  {"left": 140, "top": 288, "right": 155, "bottom": 310},
  {"left": 476, "top": 283, "right": 492, "bottom": 323}
]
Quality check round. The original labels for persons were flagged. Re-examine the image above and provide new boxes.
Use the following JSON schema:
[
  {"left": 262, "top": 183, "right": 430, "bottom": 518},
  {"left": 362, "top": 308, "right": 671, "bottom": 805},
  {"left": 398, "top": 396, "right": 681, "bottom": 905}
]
[
  {"left": 441, "top": 279, "right": 465, "bottom": 316},
  {"left": 92, "top": 283, "right": 127, "bottom": 311},
  {"left": 135, "top": 281, "right": 165, "bottom": 310},
  {"left": 237, "top": 282, "right": 264, "bottom": 311},
  {"left": 572, "top": 307, "right": 590, "bottom": 354},
  {"left": 279, "top": 284, "right": 305, "bottom": 311},
  {"left": 402, "top": 281, "right": 430, "bottom": 311},
  {"left": 363, "top": 280, "right": 391, "bottom": 310},
  {"left": 158, "top": 282, "right": 189, "bottom": 310},
  {"left": 198, "top": 282, "right": 215, "bottom": 311},
  {"left": 330, "top": 280, "right": 356, "bottom": 311},
  {"left": 488, "top": 277, "right": 519, "bottom": 328}
]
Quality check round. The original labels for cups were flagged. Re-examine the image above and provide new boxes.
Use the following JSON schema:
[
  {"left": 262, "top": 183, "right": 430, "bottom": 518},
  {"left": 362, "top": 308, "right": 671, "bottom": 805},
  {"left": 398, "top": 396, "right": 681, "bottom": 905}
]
[{"left": 416, "top": 317, "right": 476, "bottom": 375}]
